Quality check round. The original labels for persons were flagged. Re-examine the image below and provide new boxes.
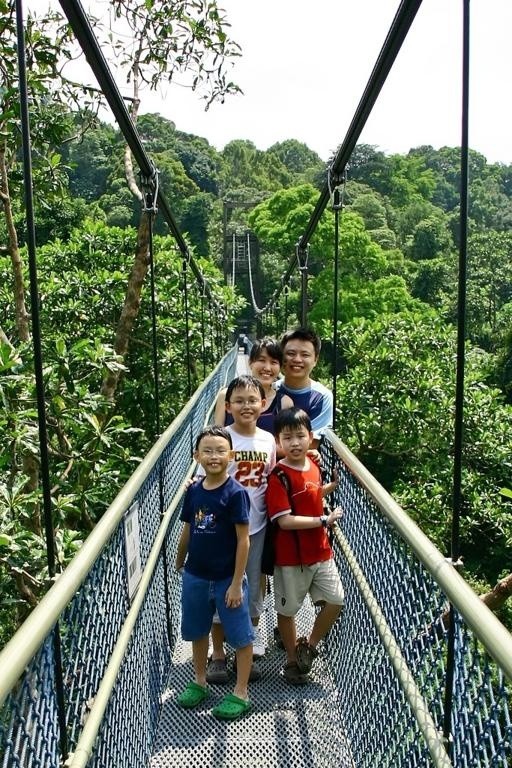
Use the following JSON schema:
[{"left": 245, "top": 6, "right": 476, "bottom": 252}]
[
  {"left": 242, "top": 333, "right": 249, "bottom": 354},
  {"left": 184, "top": 373, "right": 278, "bottom": 685},
  {"left": 272, "top": 327, "right": 334, "bottom": 450},
  {"left": 174, "top": 424, "right": 257, "bottom": 720},
  {"left": 214, "top": 336, "right": 295, "bottom": 438},
  {"left": 266, "top": 405, "right": 345, "bottom": 686}
]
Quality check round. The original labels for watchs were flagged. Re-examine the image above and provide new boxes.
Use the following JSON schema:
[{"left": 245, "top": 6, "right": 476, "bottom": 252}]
[{"left": 319, "top": 515, "right": 329, "bottom": 528}]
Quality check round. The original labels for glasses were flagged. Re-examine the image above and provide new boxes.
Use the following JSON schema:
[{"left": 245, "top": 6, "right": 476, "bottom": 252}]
[
  {"left": 199, "top": 449, "right": 228, "bottom": 458},
  {"left": 231, "top": 399, "right": 260, "bottom": 407}
]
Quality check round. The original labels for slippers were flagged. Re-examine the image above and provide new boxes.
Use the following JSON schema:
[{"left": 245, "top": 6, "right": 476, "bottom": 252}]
[
  {"left": 214, "top": 695, "right": 252, "bottom": 718},
  {"left": 178, "top": 682, "right": 209, "bottom": 708}
]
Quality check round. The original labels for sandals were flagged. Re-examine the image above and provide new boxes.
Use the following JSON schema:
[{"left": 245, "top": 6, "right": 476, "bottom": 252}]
[
  {"left": 207, "top": 655, "right": 228, "bottom": 682},
  {"left": 297, "top": 641, "right": 319, "bottom": 674},
  {"left": 232, "top": 656, "right": 262, "bottom": 679},
  {"left": 284, "top": 662, "right": 308, "bottom": 685}
]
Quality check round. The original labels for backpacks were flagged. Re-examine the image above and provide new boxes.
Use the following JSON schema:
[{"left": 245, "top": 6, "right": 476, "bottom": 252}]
[{"left": 261, "top": 458, "right": 328, "bottom": 576}]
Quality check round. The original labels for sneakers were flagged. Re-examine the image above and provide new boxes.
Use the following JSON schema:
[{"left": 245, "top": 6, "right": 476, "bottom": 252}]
[
  {"left": 274, "top": 628, "right": 285, "bottom": 649},
  {"left": 252, "top": 632, "right": 265, "bottom": 657}
]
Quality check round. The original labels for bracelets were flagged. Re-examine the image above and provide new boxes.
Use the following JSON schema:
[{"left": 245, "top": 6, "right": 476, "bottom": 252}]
[{"left": 177, "top": 565, "right": 184, "bottom": 571}]
